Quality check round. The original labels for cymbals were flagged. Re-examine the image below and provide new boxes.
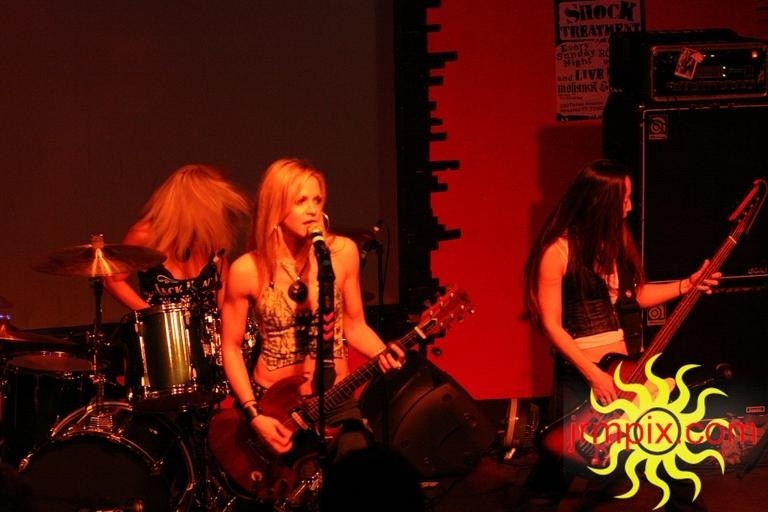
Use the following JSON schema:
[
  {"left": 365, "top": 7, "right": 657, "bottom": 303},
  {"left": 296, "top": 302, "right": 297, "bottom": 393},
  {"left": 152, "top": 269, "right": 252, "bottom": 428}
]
[
  {"left": 30, "top": 244, "right": 167, "bottom": 276},
  {"left": 0, "top": 322, "right": 73, "bottom": 346}
]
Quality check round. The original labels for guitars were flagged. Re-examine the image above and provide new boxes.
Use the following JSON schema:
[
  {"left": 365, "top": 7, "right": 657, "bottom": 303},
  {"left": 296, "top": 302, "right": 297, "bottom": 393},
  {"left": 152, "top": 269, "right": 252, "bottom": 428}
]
[
  {"left": 530, "top": 179, "right": 764, "bottom": 477},
  {"left": 208, "top": 288, "right": 475, "bottom": 498}
]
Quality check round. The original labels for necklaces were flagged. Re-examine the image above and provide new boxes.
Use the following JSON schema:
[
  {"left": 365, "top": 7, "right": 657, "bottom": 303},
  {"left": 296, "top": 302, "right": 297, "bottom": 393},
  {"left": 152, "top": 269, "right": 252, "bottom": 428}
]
[
  {"left": 173, "top": 236, "right": 194, "bottom": 262},
  {"left": 277, "top": 252, "right": 311, "bottom": 304}
]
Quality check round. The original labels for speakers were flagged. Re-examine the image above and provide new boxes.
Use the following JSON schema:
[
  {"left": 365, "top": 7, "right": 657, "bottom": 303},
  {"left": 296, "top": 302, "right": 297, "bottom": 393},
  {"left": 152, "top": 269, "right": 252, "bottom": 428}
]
[{"left": 362, "top": 352, "right": 496, "bottom": 477}]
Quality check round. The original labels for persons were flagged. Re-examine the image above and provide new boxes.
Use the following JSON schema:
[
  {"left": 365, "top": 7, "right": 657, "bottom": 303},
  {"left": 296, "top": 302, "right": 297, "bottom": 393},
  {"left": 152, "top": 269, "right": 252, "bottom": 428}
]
[
  {"left": 105, "top": 163, "right": 253, "bottom": 312},
  {"left": 521, "top": 159, "right": 723, "bottom": 501},
  {"left": 219, "top": 157, "right": 407, "bottom": 456}
]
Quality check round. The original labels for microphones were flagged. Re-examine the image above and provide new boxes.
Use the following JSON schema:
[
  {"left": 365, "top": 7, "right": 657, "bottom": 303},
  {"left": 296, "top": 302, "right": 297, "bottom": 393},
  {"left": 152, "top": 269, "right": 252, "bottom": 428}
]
[{"left": 307, "top": 223, "right": 336, "bottom": 256}]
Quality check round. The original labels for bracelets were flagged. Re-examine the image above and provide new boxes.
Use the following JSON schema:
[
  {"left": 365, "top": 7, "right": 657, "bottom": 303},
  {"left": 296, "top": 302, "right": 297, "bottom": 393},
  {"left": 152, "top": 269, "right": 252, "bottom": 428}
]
[
  {"left": 678, "top": 278, "right": 683, "bottom": 296},
  {"left": 242, "top": 399, "right": 261, "bottom": 418}
]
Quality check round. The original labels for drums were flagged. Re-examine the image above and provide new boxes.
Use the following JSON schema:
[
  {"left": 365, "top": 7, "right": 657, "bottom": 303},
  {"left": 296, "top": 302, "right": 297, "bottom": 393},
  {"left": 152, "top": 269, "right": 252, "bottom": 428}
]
[
  {"left": 121, "top": 302, "right": 228, "bottom": 415},
  {"left": 6, "top": 351, "right": 102, "bottom": 429},
  {"left": 15, "top": 403, "right": 197, "bottom": 512}
]
